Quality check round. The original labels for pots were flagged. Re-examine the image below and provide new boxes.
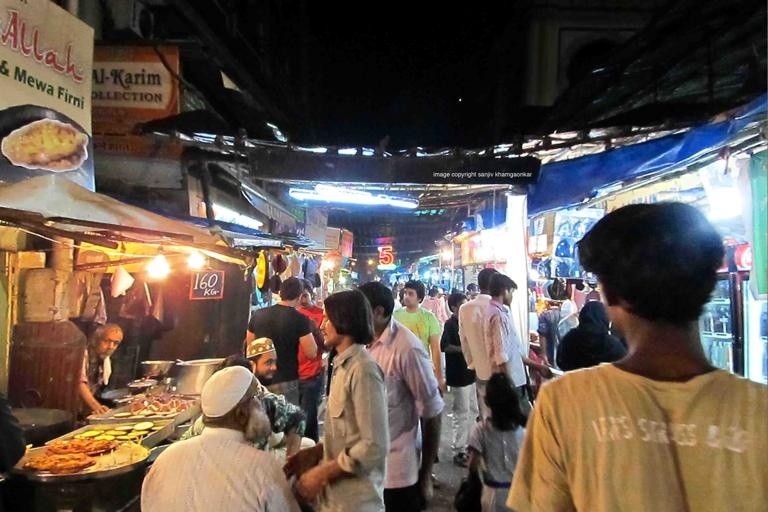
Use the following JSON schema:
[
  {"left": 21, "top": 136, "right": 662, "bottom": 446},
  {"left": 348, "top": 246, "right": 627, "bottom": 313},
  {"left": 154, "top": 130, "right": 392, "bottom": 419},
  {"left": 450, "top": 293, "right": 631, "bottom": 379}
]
[
  {"left": 0, "top": 103, "right": 94, "bottom": 190},
  {"left": 127, "top": 378, "right": 159, "bottom": 393},
  {"left": 176, "top": 359, "right": 227, "bottom": 395}
]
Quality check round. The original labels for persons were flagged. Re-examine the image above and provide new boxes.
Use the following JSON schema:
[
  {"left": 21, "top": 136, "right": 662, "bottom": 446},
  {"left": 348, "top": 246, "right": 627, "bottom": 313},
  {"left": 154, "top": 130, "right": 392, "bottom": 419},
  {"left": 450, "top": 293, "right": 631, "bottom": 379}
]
[
  {"left": 0, "top": 390, "right": 27, "bottom": 473},
  {"left": 141, "top": 200, "right": 766, "bottom": 509},
  {"left": 77, "top": 322, "right": 123, "bottom": 415}
]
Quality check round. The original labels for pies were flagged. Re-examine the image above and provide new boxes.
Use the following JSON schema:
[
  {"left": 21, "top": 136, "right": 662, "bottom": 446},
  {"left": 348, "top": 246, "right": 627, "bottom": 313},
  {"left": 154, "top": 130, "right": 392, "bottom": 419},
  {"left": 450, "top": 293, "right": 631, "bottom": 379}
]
[{"left": 22, "top": 421, "right": 164, "bottom": 470}]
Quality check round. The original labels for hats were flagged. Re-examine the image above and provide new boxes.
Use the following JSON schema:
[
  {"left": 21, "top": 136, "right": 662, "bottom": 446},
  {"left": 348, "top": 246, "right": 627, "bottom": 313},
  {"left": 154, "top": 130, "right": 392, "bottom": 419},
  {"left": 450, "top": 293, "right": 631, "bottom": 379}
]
[
  {"left": 199, "top": 365, "right": 263, "bottom": 418},
  {"left": 245, "top": 337, "right": 276, "bottom": 359}
]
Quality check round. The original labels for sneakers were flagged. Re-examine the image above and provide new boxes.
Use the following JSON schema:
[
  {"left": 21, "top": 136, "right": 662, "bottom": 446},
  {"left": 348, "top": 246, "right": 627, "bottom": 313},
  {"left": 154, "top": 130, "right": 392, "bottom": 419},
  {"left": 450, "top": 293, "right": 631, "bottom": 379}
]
[
  {"left": 431, "top": 472, "right": 441, "bottom": 486},
  {"left": 453, "top": 453, "right": 469, "bottom": 468}
]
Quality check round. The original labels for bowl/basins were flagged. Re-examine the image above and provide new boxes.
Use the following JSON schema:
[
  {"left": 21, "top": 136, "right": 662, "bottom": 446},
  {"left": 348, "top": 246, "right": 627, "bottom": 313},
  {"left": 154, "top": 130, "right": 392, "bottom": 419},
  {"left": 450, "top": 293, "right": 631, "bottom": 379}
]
[{"left": 140, "top": 359, "right": 174, "bottom": 377}]
